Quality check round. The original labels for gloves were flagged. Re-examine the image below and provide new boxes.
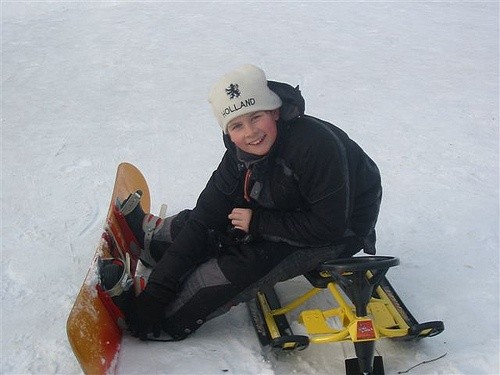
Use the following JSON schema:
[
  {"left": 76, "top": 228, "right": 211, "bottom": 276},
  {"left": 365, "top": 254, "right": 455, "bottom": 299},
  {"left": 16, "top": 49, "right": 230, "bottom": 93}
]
[{"left": 127, "top": 282, "right": 172, "bottom": 340}]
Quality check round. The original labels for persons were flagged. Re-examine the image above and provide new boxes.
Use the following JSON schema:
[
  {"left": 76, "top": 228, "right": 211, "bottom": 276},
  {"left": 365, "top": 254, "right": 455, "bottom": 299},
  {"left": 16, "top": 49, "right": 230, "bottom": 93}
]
[{"left": 105, "top": 63, "right": 383, "bottom": 340}]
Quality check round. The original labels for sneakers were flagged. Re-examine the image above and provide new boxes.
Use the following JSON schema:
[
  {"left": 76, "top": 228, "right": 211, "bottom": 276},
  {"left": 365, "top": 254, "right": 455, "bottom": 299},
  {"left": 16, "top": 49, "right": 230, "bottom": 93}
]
[
  {"left": 99, "top": 257, "right": 156, "bottom": 341},
  {"left": 118, "top": 189, "right": 167, "bottom": 268}
]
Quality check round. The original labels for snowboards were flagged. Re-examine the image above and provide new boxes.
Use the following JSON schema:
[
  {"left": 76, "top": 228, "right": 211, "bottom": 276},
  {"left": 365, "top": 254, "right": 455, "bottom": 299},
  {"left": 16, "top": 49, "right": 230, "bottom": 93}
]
[{"left": 66, "top": 163, "right": 151, "bottom": 375}]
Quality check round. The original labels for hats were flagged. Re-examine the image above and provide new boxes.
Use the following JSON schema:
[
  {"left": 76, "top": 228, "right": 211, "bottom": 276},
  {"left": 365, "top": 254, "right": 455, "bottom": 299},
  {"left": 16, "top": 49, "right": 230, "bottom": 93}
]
[{"left": 207, "top": 63, "right": 282, "bottom": 135}]
